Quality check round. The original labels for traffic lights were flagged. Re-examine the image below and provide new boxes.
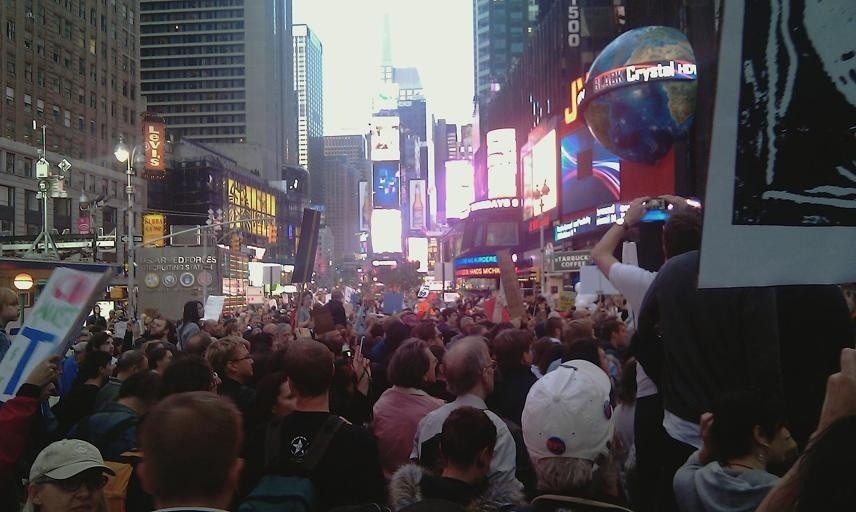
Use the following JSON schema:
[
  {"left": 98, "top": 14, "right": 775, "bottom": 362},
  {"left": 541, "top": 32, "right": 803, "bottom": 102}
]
[
  {"left": 232, "top": 236, "right": 241, "bottom": 252},
  {"left": 269, "top": 226, "right": 279, "bottom": 243}
]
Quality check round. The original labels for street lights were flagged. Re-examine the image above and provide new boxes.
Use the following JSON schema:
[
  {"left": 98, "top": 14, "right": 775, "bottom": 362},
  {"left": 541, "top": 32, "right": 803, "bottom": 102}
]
[
  {"left": 13, "top": 273, "right": 35, "bottom": 329},
  {"left": 113, "top": 133, "right": 149, "bottom": 320},
  {"left": 532, "top": 181, "right": 551, "bottom": 295},
  {"left": 78, "top": 190, "right": 107, "bottom": 236},
  {"left": 438, "top": 214, "right": 478, "bottom": 301}
]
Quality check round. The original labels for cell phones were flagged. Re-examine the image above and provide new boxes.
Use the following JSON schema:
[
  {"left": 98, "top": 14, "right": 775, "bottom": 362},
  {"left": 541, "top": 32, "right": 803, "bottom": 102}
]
[{"left": 360, "top": 336, "right": 365, "bottom": 353}]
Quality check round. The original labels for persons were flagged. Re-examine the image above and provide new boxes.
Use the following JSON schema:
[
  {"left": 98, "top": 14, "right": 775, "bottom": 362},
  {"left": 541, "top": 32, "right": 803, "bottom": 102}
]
[{"left": 1, "top": 194, "right": 856, "bottom": 511}]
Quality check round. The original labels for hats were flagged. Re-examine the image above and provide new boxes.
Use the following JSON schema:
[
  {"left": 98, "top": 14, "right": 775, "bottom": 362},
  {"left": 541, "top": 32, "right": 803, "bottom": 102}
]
[
  {"left": 29, "top": 438, "right": 117, "bottom": 482},
  {"left": 520, "top": 357, "right": 616, "bottom": 473}
]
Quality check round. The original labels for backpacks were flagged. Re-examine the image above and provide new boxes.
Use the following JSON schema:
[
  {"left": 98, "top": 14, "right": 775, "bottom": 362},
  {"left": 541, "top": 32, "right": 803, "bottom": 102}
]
[{"left": 237, "top": 411, "right": 348, "bottom": 512}]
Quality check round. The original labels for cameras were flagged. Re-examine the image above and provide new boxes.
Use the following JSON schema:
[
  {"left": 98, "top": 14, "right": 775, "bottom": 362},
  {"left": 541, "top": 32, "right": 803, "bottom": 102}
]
[
  {"left": 646, "top": 198, "right": 668, "bottom": 210},
  {"left": 131, "top": 316, "right": 138, "bottom": 324}
]
